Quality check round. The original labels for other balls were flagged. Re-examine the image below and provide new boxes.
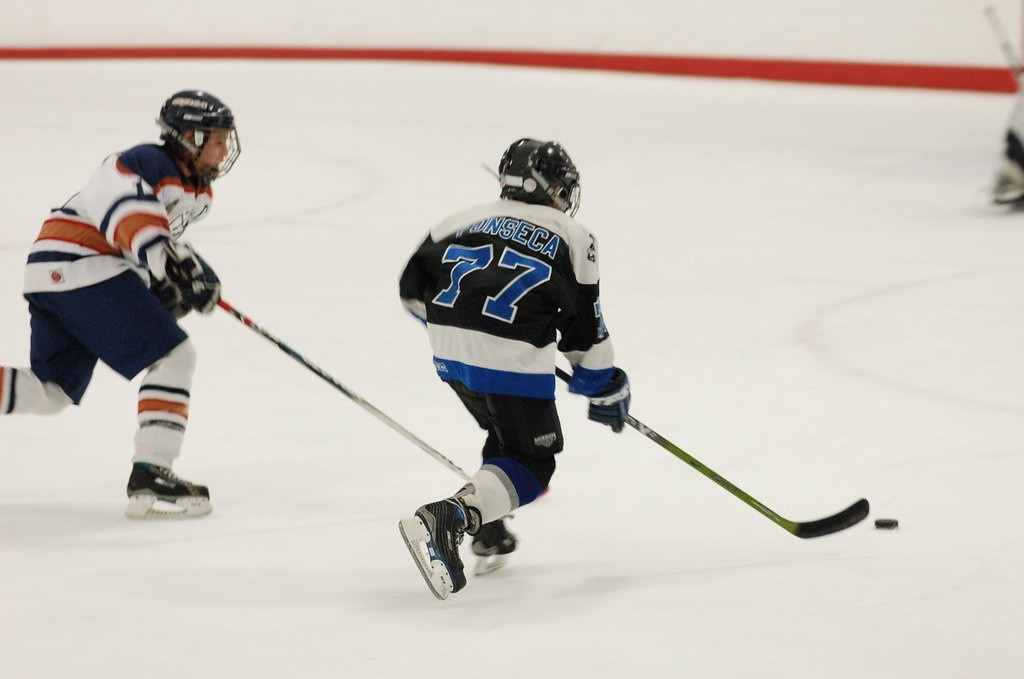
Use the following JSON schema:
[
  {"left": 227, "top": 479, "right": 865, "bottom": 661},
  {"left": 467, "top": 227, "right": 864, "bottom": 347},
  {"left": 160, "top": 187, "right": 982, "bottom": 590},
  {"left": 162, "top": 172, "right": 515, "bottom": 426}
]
[{"left": 874, "top": 518, "right": 899, "bottom": 530}]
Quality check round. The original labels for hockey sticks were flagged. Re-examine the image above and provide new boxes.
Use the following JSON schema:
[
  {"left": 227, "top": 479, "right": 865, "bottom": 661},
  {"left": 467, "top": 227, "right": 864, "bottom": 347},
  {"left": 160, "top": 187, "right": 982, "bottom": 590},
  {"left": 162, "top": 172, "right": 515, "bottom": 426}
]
[
  {"left": 981, "top": 4, "right": 1022, "bottom": 83},
  {"left": 554, "top": 366, "right": 873, "bottom": 541},
  {"left": 165, "top": 264, "right": 553, "bottom": 501}
]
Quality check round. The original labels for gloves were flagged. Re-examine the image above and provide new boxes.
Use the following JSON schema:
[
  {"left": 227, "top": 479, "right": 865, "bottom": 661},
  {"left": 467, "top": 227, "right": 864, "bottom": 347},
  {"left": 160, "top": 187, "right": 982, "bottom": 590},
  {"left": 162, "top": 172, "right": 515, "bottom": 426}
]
[
  {"left": 588, "top": 369, "right": 631, "bottom": 432},
  {"left": 172, "top": 248, "right": 222, "bottom": 313},
  {"left": 147, "top": 278, "right": 191, "bottom": 318}
]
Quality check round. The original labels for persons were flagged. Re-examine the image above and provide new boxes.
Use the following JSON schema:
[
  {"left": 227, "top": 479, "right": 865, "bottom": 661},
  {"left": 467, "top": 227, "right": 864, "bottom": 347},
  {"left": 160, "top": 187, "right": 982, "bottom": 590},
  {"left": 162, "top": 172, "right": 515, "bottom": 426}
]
[
  {"left": 396, "top": 138, "right": 631, "bottom": 600},
  {"left": 994, "top": 74, "right": 1024, "bottom": 205},
  {"left": 1, "top": 91, "right": 242, "bottom": 520}
]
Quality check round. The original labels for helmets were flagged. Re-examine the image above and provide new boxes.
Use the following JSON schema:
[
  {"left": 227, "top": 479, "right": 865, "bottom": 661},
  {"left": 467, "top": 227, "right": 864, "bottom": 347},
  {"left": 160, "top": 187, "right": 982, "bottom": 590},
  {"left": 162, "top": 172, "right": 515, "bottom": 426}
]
[
  {"left": 157, "top": 91, "right": 241, "bottom": 184},
  {"left": 498, "top": 138, "right": 581, "bottom": 217}
]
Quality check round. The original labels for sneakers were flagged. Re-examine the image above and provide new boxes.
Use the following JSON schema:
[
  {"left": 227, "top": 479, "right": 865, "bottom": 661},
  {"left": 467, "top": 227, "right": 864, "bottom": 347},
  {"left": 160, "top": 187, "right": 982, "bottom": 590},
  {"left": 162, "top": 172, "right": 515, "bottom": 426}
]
[
  {"left": 471, "top": 515, "right": 518, "bottom": 576},
  {"left": 399, "top": 484, "right": 482, "bottom": 602},
  {"left": 125, "top": 464, "right": 213, "bottom": 522}
]
